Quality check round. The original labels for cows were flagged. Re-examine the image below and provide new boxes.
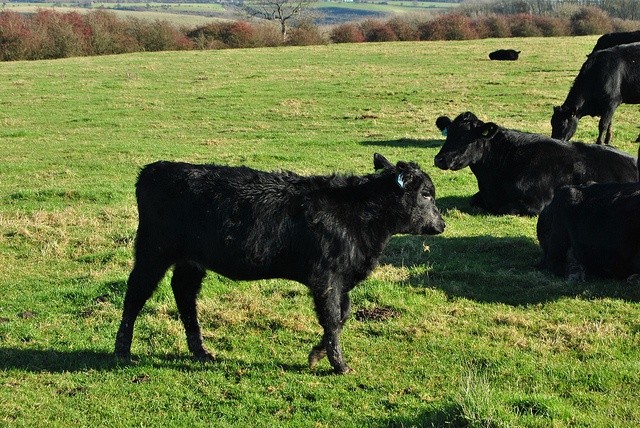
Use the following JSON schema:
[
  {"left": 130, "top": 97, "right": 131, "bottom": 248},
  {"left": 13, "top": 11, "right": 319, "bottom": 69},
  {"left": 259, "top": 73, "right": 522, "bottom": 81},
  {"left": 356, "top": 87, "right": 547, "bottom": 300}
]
[
  {"left": 551, "top": 42, "right": 640, "bottom": 144},
  {"left": 112, "top": 150, "right": 447, "bottom": 376},
  {"left": 536, "top": 177, "right": 640, "bottom": 283},
  {"left": 586, "top": 29, "right": 640, "bottom": 58},
  {"left": 489, "top": 49, "right": 521, "bottom": 60},
  {"left": 434, "top": 110, "right": 640, "bottom": 215}
]
